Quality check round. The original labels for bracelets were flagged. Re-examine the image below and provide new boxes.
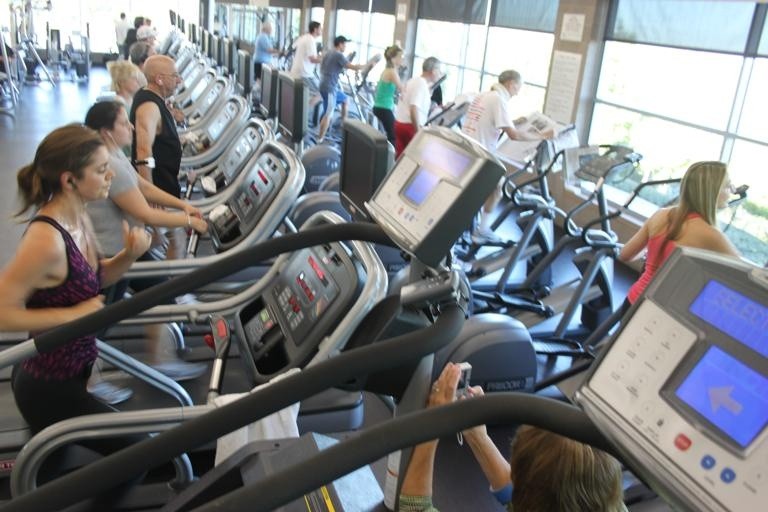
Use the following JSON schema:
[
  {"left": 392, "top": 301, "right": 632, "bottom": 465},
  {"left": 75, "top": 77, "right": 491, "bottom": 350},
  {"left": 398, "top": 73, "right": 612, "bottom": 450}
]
[{"left": 183, "top": 214, "right": 191, "bottom": 230}]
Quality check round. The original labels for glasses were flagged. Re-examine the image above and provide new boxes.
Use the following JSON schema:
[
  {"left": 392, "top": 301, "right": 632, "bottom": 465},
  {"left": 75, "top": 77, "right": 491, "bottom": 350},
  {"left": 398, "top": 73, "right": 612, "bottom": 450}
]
[{"left": 160, "top": 73, "right": 180, "bottom": 79}]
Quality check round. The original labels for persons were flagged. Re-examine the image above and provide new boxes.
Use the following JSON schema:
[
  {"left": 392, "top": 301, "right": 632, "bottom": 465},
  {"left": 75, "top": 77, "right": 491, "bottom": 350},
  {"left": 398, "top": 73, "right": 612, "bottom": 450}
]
[
  {"left": 619, "top": 162, "right": 743, "bottom": 322},
  {"left": 394, "top": 58, "right": 441, "bottom": 159},
  {"left": 110, "top": 64, "right": 142, "bottom": 120},
  {"left": 132, "top": 42, "right": 155, "bottom": 67},
  {"left": 132, "top": 56, "right": 182, "bottom": 259},
  {"left": 2, "top": 125, "right": 198, "bottom": 487},
  {"left": 0, "top": 39, "right": 13, "bottom": 91},
  {"left": 82, "top": 102, "right": 208, "bottom": 404},
  {"left": 124, "top": 19, "right": 143, "bottom": 58},
  {"left": 292, "top": 20, "right": 323, "bottom": 107},
  {"left": 401, "top": 364, "right": 630, "bottom": 512},
  {"left": 316, "top": 35, "right": 366, "bottom": 145},
  {"left": 252, "top": 22, "right": 287, "bottom": 92},
  {"left": 462, "top": 70, "right": 557, "bottom": 247},
  {"left": 374, "top": 45, "right": 408, "bottom": 145},
  {"left": 138, "top": 27, "right": 157, "bottom": 50},
  {"left": 116, "top": 12, "right": 130, "bottom": 56}
]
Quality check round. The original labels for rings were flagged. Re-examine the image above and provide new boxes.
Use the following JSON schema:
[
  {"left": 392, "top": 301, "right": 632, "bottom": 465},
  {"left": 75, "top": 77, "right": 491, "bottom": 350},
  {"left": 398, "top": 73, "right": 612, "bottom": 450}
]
[{"left": 433, "top": 387, "right": 439, "bottom": 394}]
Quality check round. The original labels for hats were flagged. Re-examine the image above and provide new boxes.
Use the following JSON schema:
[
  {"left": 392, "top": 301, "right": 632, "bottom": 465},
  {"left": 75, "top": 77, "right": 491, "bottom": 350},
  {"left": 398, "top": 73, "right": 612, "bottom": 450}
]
[{"left": 334, "top": 36, "right": 351, "bottom": 44}]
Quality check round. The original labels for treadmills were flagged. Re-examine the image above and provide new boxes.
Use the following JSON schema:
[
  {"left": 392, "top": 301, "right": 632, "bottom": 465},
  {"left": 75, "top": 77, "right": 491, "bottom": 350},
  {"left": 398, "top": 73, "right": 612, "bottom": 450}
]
[
  {"left": 175, "top": 70, "right": 217, "bottom": 112},
  {"left": 104, "top": 141, "right": 305, "bottom": 301},
  {"left": 178, "top": 116, "right": 276, "bottom": 207},
  {"left": 176, "top": 77, "right": 235, "bottom": 128},
  {"left": 179, "top": 96, "right": 251, "bottom": 163},
  {"left": 175, "top": 47, "right": 193, "bottom": 66},
  {"left": 1, "top": 124, "right": 506, "bottom": 511},
  {"left": 194, "top": 245, "right": 768, "bottom": 511},
  {"left": 172, "top": 61, "right": 208, "bottom": 97},
  {"left": 0, "top": 210, "right": 387, "bottom": 498},
  {"left": 180, "top": 54, "right": 200, "bottom": 76}
]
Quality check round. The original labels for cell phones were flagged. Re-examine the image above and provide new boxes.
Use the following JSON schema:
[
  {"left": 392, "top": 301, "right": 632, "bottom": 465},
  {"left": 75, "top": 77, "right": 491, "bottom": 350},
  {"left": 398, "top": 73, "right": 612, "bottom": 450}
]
[{"left": 452, "top": 362, "right": 472, "bottom": 399}]
